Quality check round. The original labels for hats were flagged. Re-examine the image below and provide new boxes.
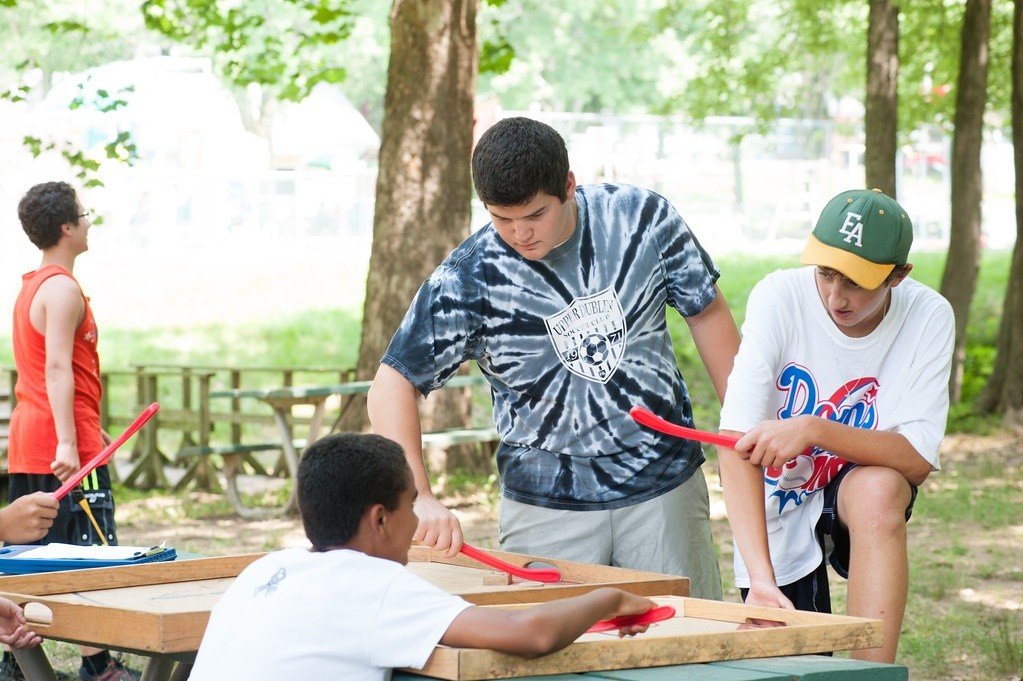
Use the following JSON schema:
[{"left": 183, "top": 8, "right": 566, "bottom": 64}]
[{"left": 801, "top": 188, "right": 913, "bottom": 290}]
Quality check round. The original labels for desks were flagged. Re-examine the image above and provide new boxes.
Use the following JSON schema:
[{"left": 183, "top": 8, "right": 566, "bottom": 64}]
[
  {"left": 206, "top": 376, "right": 485, "bottom": 518},
  {"left": 10, "top": 633, "right": 197, "bottom": 680}
]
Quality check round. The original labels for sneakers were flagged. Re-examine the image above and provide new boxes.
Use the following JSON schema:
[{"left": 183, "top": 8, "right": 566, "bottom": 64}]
[
  {"left": 80, "top": 652, "right": 142, "bottom": 681},
  {"left": 0, "top": 658, "right": 74, "bottom": 681}
]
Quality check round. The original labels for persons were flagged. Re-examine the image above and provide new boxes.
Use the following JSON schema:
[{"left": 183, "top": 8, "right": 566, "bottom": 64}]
[
  {"left": 188, "top": 432, "right": 659, "bottom": 681},
  {"left": 366, "top": 117, "right": 745, "bottom": 599},
  {"left": 716, "top": 187, "right": 955, "bottom": 666},
  {"left": 0, "top": 181, "right": 142, "bottom": 681}
]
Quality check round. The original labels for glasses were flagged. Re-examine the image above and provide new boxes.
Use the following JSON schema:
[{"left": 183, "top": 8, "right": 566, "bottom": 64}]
[{"left": 65, "top": 212, "right": 89, "bottom": 222}]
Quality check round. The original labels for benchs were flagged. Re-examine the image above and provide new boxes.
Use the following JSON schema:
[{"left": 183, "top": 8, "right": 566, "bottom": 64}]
[
  {"left": 182, "top": 441, "right": 303, "bottom": 511},
  {"left": 421, "top": 429, "right": 501, "bottom": 474}
]
